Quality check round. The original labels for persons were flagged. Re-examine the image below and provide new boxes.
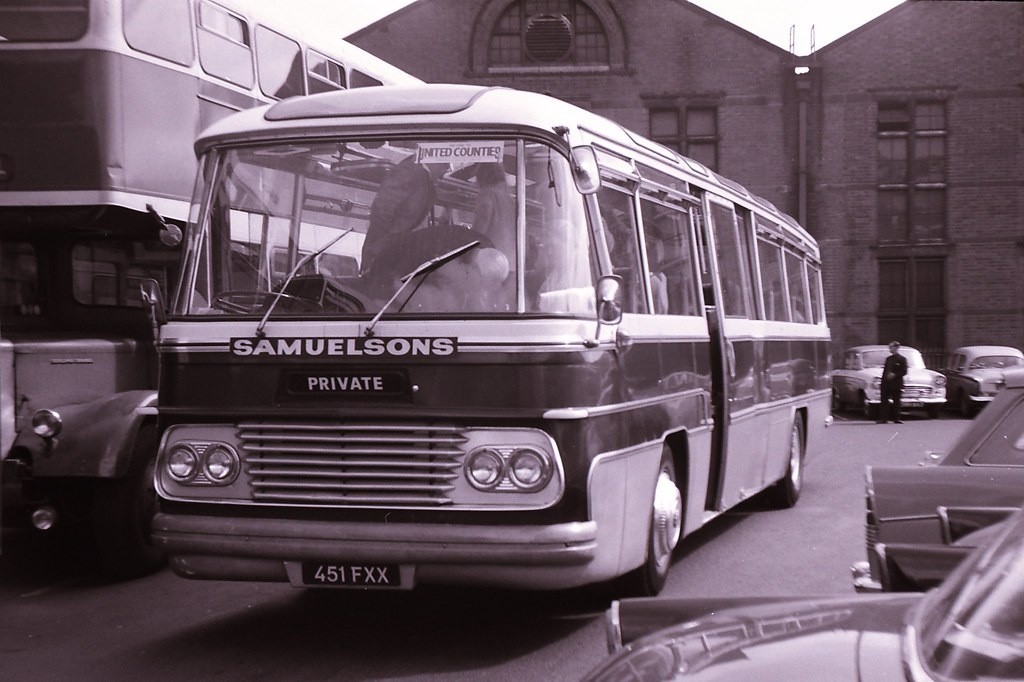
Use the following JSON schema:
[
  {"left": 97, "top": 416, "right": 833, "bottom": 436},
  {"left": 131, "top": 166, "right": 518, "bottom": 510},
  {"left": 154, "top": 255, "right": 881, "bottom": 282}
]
[
  {"left": 362, "top": 155, "right": 451, "bottom": 273},
  {"left": 617, "top": 220, "right": 668, "bottom": 315},
  {"left": 876, "top": 341, "right": 908, "bottom": 424},
  {"left": 534, "top": 179, "right": 616, "bottom": 293},
  {"left": 472, "top": 162, "right": 527, "bottom": 312}
]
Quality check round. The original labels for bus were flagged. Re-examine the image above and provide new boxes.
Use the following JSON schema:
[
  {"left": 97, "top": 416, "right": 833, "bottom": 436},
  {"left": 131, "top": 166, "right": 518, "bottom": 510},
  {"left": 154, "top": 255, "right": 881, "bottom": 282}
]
[
  {"left": 0, "top": 1, "right": 538, "bottom": 576},
  {"left": 139, "top": 85, "right": 833, "bottom": 604}
]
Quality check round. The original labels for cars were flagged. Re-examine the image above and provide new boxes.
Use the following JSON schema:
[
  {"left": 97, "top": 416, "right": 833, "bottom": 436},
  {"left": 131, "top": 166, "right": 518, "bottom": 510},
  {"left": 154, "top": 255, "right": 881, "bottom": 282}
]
[
  {"left": 581, "top": 505, "right": 1024, "bottom": 682},
  {"left": 833, "top": 345, "right": 947, "bottom": 420},
  {"left": 850, "top": 370, "right": 1024, "bottom": 593},
  {"left": 937, "top": 345, "right": 1024, "bottom": 418}
]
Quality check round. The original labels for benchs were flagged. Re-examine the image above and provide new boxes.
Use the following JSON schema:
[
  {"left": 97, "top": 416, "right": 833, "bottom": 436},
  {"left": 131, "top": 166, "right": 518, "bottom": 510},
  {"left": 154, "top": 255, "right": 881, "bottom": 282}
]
[{"left": 665, "top": 275, "right": 821, "bottom": 325}]
